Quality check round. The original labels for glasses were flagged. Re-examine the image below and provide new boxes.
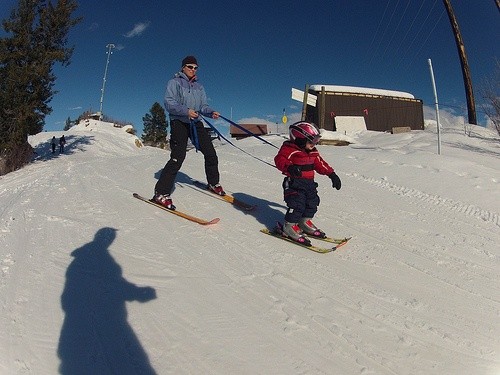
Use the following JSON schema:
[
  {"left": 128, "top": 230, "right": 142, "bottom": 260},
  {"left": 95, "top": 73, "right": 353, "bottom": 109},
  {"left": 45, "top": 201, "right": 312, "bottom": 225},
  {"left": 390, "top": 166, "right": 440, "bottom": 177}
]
[{"left": 186, "top": 65, "right": 198, "bottom": 70}]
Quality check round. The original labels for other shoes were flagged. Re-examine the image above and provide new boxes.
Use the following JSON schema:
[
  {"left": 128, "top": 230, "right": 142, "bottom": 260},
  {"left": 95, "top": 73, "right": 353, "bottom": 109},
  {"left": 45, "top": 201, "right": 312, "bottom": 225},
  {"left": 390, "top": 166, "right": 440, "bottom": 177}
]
[
  {"left": 154, "top": 190, "right": 176, "bottom": 210},
  {"left": 283, "top": 221, "right": 309, "bottom": 244},
  {"left": 208, "top": 182, "right": 226, "bottom": 196},
  {"left": 301, "top": 221, "right": 324, "bottom": 236}
]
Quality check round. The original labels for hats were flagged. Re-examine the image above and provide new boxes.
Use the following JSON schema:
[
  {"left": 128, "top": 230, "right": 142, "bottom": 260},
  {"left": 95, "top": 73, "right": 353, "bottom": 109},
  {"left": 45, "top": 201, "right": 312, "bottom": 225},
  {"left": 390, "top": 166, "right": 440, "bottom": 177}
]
[{"left": 182, "top": 56, "right": 196, "bottom": 66}]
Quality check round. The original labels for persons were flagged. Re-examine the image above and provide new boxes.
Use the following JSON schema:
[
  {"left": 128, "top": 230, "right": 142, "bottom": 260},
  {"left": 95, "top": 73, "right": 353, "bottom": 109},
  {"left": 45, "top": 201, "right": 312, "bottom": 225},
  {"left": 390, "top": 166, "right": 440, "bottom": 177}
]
[
  {"left": 51, "top": 136, "right": 56, "bottom": 153},
  {"left": 274, "top": 121, "right": 341, "bottom": 240},
  {"left": 59, "top": 135, "right": 65, "bottom": 152},
  {"left": 153, "top": 56, "right": 226, "bottom": 207}
]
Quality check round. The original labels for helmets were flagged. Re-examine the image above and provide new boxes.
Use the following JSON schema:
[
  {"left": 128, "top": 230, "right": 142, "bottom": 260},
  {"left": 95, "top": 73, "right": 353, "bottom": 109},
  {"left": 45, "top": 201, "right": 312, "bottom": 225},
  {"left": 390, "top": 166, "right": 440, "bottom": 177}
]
[{"left": 289, "top": 122, "right": 319, "bottom": 142}]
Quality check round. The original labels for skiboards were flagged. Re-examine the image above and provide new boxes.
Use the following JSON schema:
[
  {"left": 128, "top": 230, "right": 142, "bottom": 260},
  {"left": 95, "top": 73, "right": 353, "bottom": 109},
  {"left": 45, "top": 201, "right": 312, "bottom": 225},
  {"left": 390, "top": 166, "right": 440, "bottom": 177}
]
[
  {"left": 132, "top": 177, "right": 256, "bottom": 226},
  {"left": 260, "top": 226, "right": 353, "bottom": 254}
]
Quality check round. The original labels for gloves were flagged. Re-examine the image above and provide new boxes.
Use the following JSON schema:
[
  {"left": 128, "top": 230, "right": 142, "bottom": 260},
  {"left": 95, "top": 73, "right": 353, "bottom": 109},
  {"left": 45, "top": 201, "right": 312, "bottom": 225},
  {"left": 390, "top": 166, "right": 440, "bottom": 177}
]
[
  {"left": 329, "top": 172, "right": 342, "bottom": 190},
  {"left": 288, "top": 165, "right": 301, "bottom": 176}
]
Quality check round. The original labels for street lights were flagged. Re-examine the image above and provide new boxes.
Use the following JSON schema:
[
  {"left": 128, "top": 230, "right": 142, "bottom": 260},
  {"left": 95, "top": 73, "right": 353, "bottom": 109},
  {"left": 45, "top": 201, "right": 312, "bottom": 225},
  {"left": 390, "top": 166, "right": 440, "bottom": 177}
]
[{"left": 99, "top": 43, "right": 116, "bottom": 121}]
